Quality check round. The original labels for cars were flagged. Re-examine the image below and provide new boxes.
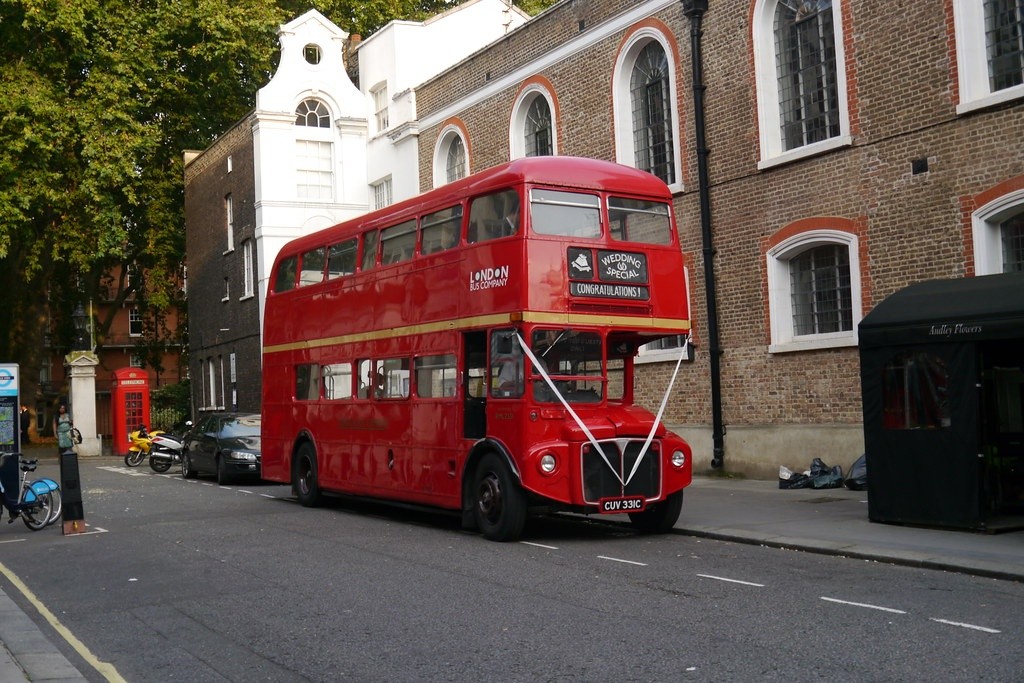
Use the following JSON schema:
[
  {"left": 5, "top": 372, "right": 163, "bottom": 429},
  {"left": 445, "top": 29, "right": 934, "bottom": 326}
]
[{"left": 181, "top": 412, "right": 262, "bottom": 486}]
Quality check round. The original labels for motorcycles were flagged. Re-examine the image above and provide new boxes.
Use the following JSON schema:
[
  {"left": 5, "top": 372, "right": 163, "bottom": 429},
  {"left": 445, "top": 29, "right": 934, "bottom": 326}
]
[{"left": 123, "top": 418, "right": 193, "bottom": 474}]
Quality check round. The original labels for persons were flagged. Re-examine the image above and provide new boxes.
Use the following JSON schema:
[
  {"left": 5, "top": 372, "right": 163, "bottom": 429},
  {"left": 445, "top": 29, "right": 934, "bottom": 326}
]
[
  {"left": 51, "top": 403, "right": 74, "bottom": 453},
  {"left": 377, "top": 373, "right": 383, "bottom": 395},
  {"left": 494, "top": 207, "right": 517, "bottom": 237},
  {"left": 498, "top": 355, "right": 550, "bottom": 392},
  {"left": 19, "top": 404, "right": 31, "bottom": 445}
]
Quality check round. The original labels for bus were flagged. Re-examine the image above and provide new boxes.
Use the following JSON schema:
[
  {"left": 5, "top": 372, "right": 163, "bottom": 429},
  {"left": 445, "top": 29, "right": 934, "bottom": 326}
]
[{"left": 258, "top": 154, "right": 696, "bottom": 542}]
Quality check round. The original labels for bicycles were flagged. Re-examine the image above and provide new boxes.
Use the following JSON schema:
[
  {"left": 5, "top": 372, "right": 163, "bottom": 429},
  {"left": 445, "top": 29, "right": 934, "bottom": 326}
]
[{"left": 0, "top": 451, "right": 63, "bottom": 531}]
